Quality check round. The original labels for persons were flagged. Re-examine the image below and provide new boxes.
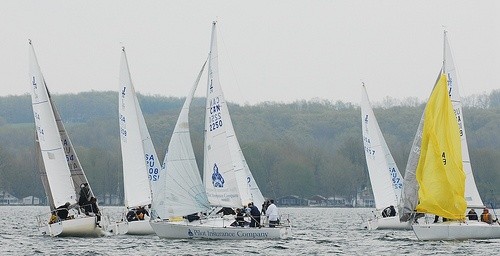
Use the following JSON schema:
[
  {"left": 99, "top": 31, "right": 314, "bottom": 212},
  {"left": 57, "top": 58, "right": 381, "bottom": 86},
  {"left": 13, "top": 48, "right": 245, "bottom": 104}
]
[
  {"left": 215, "top": 199, "right": 278, "bottom": 229},
  {"left": 50, "top": 183, "right": 101, "bottom": 229},
  {"left": 121, "top": 203, "right": 153, "bottom": 223},
  {"left": 382, "top": 205, "right": 397, "bottom": 219},
  {"left": 433, "top": 208, "right": 497, "bottom": 224}
]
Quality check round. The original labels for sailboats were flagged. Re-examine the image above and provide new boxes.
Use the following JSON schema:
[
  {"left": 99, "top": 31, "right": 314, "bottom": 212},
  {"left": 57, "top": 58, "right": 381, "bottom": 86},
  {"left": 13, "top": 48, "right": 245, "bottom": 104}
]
[
  {"left": 27, "top": 19, "right": 298, "bottom": 240},
  {"left": 361, "top": 30, "right": 500, "bottom": 242}
]
